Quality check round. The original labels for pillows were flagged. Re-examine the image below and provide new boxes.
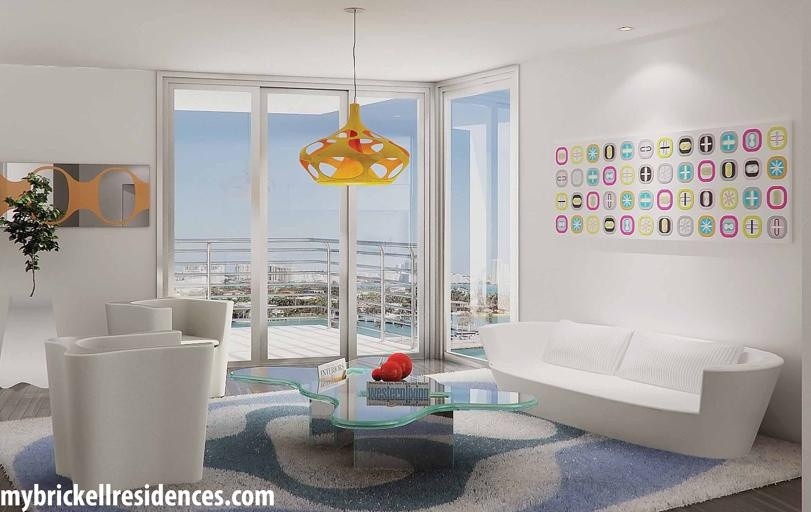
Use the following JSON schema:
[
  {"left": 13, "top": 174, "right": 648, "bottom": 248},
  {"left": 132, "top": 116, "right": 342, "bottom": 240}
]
[{"left": 540, "top": 317, "right": 743, "bottom": 398}]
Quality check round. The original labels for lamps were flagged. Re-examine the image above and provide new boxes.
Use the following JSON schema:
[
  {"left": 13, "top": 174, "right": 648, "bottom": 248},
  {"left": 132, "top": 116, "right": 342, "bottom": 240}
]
[{"left": 300, "top": 5, "right": 410, "bottom": 184}]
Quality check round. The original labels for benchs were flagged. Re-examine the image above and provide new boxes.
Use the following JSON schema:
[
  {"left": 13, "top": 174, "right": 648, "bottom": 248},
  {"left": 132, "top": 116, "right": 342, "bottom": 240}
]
[{"left": 476, "top": 317, "right": 784, "bottom": 461}]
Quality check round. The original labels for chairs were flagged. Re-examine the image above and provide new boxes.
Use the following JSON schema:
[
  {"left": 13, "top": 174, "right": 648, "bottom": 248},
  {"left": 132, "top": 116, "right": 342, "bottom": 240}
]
[{"left": 42, "top": 295, "right": 232, "bottom": 500}]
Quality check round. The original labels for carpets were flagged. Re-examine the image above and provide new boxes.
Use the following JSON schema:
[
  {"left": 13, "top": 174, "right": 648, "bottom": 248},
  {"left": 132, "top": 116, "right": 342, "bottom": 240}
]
[{"left": 0, "top": 366, "right": 799, "bottom": 511}]
